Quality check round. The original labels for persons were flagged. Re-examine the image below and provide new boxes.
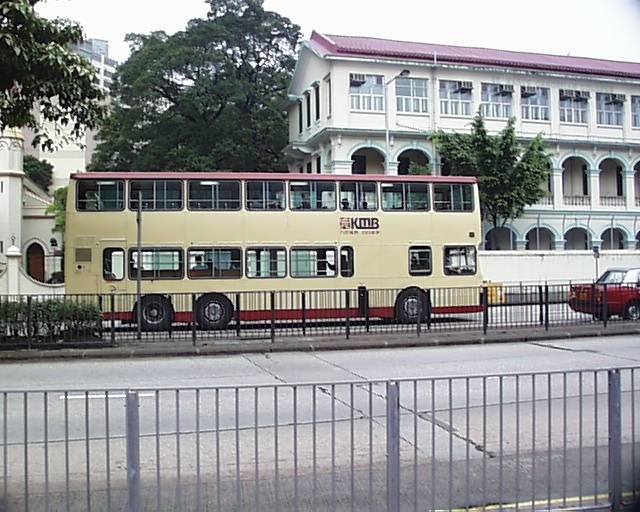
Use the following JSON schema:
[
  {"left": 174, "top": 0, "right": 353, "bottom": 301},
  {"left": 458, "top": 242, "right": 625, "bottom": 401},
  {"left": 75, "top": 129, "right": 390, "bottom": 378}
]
[
  {"left": 192, "top": 255, "right": 206, "bottom": 270},
  {"left": 327, "top": 255, "right": 348, "bottom": 277}
]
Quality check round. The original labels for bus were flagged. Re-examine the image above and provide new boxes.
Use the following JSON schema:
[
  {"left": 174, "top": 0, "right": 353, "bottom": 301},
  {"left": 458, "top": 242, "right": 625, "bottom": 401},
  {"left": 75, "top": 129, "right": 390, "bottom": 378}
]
[{"left": 64, "top": 172, "right": 487, "bottom": 332}]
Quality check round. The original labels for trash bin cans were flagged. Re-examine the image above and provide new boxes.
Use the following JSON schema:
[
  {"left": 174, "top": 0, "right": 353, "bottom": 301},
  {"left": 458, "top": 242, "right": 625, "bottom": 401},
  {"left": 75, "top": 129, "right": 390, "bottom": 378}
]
[{"left": 488, "top": 283, "right": 506, "bottom": 304}]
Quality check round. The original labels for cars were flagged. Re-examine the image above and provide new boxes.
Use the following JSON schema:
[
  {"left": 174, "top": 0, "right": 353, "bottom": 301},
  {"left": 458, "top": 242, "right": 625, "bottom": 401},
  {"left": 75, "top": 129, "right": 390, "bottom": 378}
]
[{"left": 569, "top": 268, "right": 640, "bottom": 322}]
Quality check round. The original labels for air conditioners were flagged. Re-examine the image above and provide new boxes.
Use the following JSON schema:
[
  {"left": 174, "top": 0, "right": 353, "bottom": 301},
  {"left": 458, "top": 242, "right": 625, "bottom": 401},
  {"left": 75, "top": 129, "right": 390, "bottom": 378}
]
[
  {"left": 457, "top": 80, "right": 472, "bottom": 91},
  {"left": 498, "top": 84, "right": 513, "bottom": 94},
  {"left": 351, "top": 73, "right": 366, "bottom": 85},
  {"left": 613, "top": 94, "right": 625, "bottom": 104},
  {"left": 577, "top": 90, "right": 590, "bottom": 101},
  {"left": 559, "top": 89, "right": 575, "bottom": 99},
  {"left": 522, "top": 86, "right": 538, "bottom": 96}
]
